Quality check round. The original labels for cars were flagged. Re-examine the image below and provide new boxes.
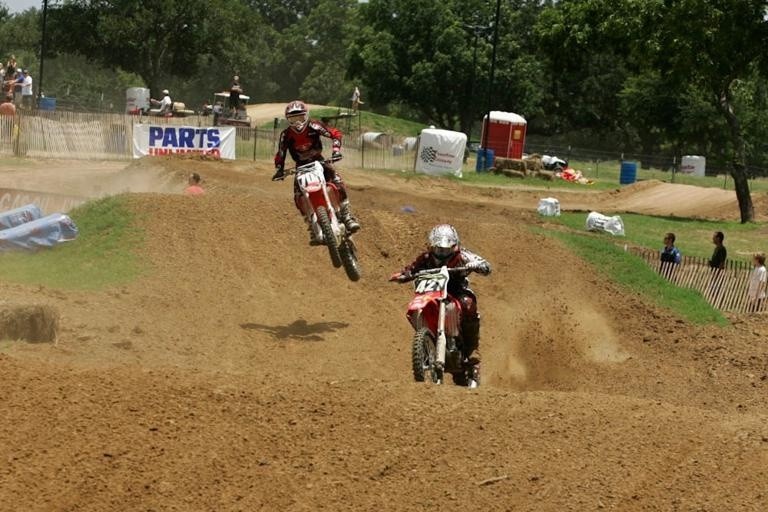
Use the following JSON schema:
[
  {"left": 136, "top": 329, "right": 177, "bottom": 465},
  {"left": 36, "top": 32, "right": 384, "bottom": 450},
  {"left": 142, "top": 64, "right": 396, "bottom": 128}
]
[
  {"left": 469, "top": 143, "right": 481, "bottom": 152},
  {"left": 522, "top": 153, "right": 568, "bottom": 173}
]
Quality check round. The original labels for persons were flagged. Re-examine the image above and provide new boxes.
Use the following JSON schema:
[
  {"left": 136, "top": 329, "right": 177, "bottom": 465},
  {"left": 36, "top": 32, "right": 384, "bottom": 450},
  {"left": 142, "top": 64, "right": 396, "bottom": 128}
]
[
  {"left": 748, "top": 252, "right": 767, "bottom": 313},
  {"left": 0, "top": 55, "right": 33, "bottom": 136},
  {"left": 392, "top": 223, "right": 492, "bottom": 364},
  {"left": 351, "top": 85, "right": 360, "bottom": 113},
  {"left": 707, "top": 232, "right": 726, "bottom": 269},
  {"left": 273, "top": 101, "right": 360, "bottom": 245},
  {"left": 227, "top": 74, "right": 243, "bottom": 121},
  {"left": 659, "top": 234, "right": 681, "bottom": 281},
  {"left": 148, "top": 88, "right": 172, "bottom": 116},
  {"left": 212, "top": 101, "right": 223, "bottom": 126},
  {"left": 184, "top": 173, "right": 205, "bottom": 194}
]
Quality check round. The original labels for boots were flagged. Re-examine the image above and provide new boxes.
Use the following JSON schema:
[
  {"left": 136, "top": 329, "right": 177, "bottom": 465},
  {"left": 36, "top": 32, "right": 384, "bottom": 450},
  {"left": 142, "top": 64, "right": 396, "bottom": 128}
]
[
  {"left": 339, "top": 199, "right": 360, "bottom": 233},
  {"left": 462, "top": 319, "right": 480, "bottom": 361},
  {"left": 305, "top": 217, "right": 317, "bottom": 245}
]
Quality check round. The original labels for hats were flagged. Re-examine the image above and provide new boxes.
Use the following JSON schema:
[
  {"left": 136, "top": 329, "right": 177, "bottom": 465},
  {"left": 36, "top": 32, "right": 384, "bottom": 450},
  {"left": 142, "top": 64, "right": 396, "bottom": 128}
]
[{"left": 163, "top": 90, "right": 169, "bottom": 94}]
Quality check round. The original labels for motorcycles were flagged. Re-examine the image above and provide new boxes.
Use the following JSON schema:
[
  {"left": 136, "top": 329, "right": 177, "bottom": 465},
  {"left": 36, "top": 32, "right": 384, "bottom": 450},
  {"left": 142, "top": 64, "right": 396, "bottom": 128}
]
[
  {"left": 272, "top": 154, "right": 360, "bottom": 282},
  {"left": 388, "top": 263, "right": 481, "bottom": 387}
]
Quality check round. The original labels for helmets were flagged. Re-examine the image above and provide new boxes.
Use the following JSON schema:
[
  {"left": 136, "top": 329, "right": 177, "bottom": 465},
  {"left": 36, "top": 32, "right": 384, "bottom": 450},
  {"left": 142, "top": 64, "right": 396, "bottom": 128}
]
[
  {"left": 285, "top": 101, "right": 309, "bottom": 134},
  {"left": 430, "top": 224, "right": 459, "bottom": 260}
]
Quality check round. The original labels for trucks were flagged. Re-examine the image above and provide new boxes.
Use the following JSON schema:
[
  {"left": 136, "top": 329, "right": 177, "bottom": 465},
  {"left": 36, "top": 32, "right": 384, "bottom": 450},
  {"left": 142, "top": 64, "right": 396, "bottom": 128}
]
[{"left": 212, "top": 91, "right": 251, "bottom": 142}]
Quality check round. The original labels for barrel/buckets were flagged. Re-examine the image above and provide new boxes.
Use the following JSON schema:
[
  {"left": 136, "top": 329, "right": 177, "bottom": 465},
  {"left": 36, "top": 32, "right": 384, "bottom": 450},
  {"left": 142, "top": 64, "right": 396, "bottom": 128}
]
[
  {"left": 682, "top": 155, "right": 696, "bottom": 175},
  {"left": 36, "top": 97, "right": 56, "bottom": 111},
  {"left": 476, "top": 149, "right": 494, "bottom": 172},
  {"left": 619, "top": 161, "right": 637, "bottom": 184},
  {"left": 689, "top": 156, "right": 706, "bottom": 176}
]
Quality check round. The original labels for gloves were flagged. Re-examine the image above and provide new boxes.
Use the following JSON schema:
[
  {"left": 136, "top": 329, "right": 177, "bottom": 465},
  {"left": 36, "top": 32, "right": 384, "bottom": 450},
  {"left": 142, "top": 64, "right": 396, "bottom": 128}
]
[
  {"left": 465, "top": 261, "right": 479, "bottom": 274},
  {"left": 332, "top": 151, "right": 342, "bottom": 164},
  {"left": 273, "top": 167, "right": 283, "bottom": 180},
  {"left": 390, "top": 272, "right": 402, "bottom": 282}
]
